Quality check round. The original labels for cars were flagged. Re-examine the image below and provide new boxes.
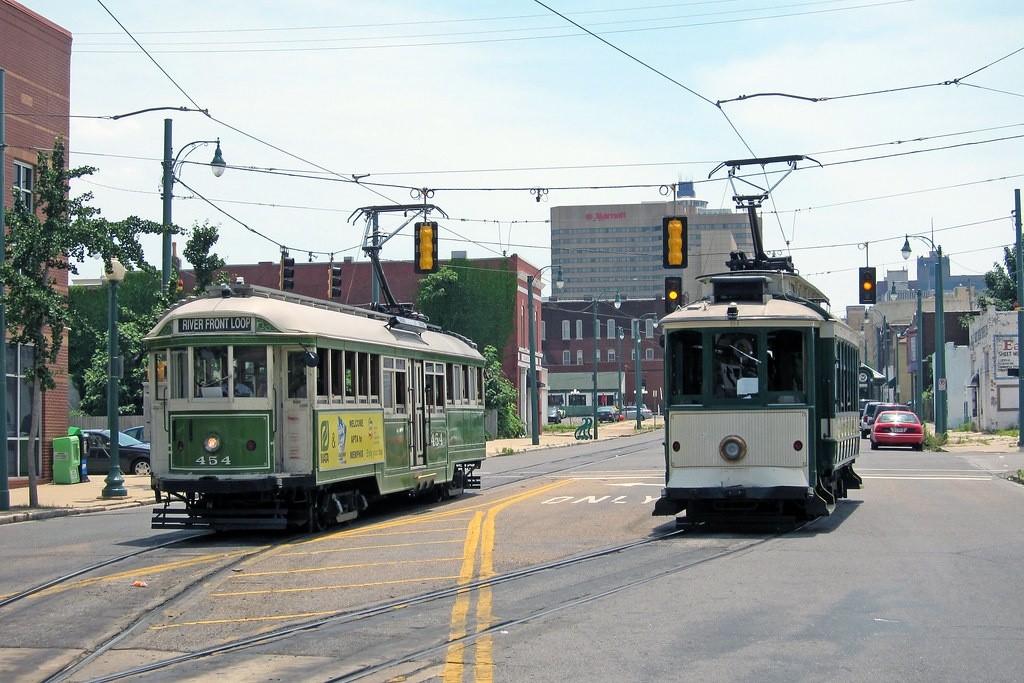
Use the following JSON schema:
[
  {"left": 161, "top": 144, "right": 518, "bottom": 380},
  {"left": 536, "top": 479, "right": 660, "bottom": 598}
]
[
  {"left": 548, "top": 404, "right": 566, "bottom": 424},
  {"left": 82, "top": 426, "right": 152, "bottom": 475},
  {"left": 597, "top": 406, "right": 619, "bottom": 423},
  {"left": 870, "top": 410, "right": 923, "bottom": 450},
  {"left": 617, "top": 405, "right": 653, "bottom": 420}
]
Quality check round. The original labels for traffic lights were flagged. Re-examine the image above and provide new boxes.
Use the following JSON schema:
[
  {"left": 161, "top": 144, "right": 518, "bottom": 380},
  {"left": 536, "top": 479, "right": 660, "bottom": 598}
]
[
  {"left": 859, "top": 267, "right": 876, "bottom": 304},
  {"left": 279, "top": 253, "right": 294, "bottom": 291},
  {"left": 665, "top": 277, "right": 682, "bottom": 313},
  {"left": 327, "top": 266, "right": 341, "bottom": 299}
]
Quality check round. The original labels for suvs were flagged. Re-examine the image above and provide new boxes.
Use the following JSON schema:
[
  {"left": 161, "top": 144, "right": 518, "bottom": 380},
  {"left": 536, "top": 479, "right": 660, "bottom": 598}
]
[{"left": 859, "top": 400, "right": 911, "bottom": 439}]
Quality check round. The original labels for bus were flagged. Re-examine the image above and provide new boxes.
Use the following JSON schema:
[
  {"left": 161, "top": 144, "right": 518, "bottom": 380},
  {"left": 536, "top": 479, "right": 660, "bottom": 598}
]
[
  {"left": 652, "top": 251, "right": 862, "bottom": 532},
  {"left": 140, "top": 284, "right": 493, "bottom": 535}
]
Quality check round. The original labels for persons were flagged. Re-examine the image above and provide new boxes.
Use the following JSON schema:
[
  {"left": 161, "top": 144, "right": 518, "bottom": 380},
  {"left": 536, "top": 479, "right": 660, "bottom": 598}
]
[
  {"left": 220, "top": 366, "right": 254, "bottom": 397},
  {"left": 256, "top": 361, "right": 316, "bottom": 400}
]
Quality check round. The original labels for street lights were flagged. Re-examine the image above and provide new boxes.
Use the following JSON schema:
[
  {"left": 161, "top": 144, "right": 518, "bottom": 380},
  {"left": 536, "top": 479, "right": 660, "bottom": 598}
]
[
  {"left": 159, "top": 119, "right": 228, "bottom": 310},
  {"left": 633, "top": 312, "right": 658, "bottom": 429},
  {"left": 900, "top": 234, "right": 947, "bottom": 442},
  {"left": 527, "top": 264, "right": 565, "bottom": 445},
  {"left": 864, "top": 280, "right": 923, "bottom": 422},
  {"left": 102, "top": 255, "right": 127, "bottom": 497},
  {"left": 592, "top": 290, "right": 622, "bottom": 439}
]
